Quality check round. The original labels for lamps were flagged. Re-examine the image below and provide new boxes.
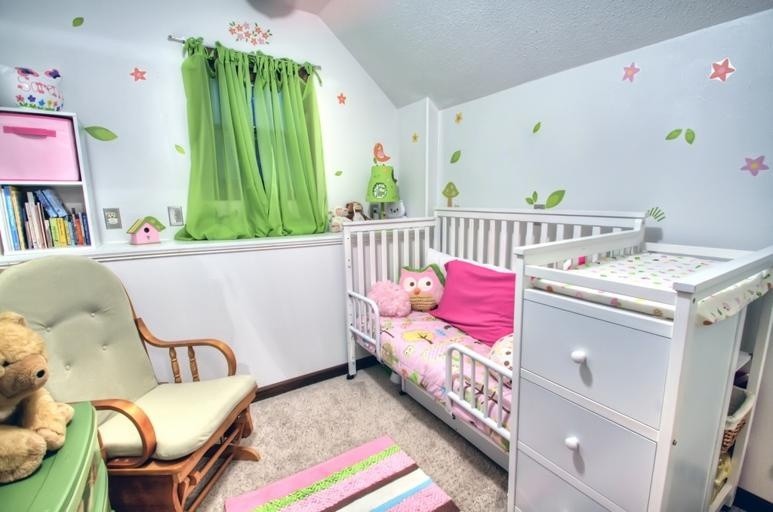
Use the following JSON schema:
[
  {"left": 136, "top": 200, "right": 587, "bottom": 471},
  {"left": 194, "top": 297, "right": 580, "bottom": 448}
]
[{"left": 366, "top": 166, "right": 400, "bottom": 218}]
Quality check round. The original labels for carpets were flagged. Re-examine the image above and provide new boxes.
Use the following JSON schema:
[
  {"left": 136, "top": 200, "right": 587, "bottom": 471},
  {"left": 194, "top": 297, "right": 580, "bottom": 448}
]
[{"left": 222, "top": 434, "right": 462, "bottom": 512}]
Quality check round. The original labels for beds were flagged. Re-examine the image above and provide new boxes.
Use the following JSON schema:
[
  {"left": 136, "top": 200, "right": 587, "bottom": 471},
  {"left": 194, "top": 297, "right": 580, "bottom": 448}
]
[{"left": 342, "top": 207, "right": 647, "bottom": 473}]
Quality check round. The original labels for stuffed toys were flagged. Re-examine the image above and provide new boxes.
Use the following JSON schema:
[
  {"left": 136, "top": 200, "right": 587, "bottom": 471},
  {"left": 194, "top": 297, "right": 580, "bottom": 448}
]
[
  {"left": 0, "top": 311, "right": 74, "bottom": 489},
  {"left": 345, "top": 202, "right": 370, "bottom": 222},
  {"left": 397, "top": 263, "right": 446, "bottom": 312},
  {"left": 367, "top": 280, "right": 411, "bottom": 316},
  {"left": 329, "top": 206, "right": 352, "bottom": 233}
]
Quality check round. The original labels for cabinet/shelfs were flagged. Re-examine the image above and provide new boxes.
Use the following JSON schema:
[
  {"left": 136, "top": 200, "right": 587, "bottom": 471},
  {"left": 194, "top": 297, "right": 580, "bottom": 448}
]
[
  {"left": 0, "top": 105, "right": 100, "bottom": 259},
  {"left": 0, "top": 402, "right": 111, "bottom": 512},
  {"left": 505, "top": 230, "right": 773, "bottom": 511}
]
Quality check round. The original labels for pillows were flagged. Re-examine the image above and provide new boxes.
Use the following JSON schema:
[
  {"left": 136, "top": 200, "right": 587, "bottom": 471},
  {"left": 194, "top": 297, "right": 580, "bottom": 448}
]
[{"left": 430, "top": 259, "right": 516, "bottom": 347}]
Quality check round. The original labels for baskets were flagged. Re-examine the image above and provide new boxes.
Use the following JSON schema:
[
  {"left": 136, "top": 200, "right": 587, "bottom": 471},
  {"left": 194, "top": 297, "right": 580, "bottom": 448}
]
[{"left": 720, "top": 386, "right": 757, "bottom": 453}]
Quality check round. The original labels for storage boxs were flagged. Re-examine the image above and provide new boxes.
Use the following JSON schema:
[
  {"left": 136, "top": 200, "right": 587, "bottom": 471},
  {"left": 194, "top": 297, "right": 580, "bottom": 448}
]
[{"left": 1, "top": 113, "right": 80, "bottom": 182}]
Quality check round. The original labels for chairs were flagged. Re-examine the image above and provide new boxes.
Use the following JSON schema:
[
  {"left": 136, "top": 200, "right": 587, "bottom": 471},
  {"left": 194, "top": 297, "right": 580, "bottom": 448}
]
[{"left": 0, "top": 255, "right": 258, "bottom": 512}]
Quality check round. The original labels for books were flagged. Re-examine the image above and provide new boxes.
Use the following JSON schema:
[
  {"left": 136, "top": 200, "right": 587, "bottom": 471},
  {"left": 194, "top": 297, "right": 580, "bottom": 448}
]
[{"left": 1, "top": 184, "right": 91, "bottom": 253}]
